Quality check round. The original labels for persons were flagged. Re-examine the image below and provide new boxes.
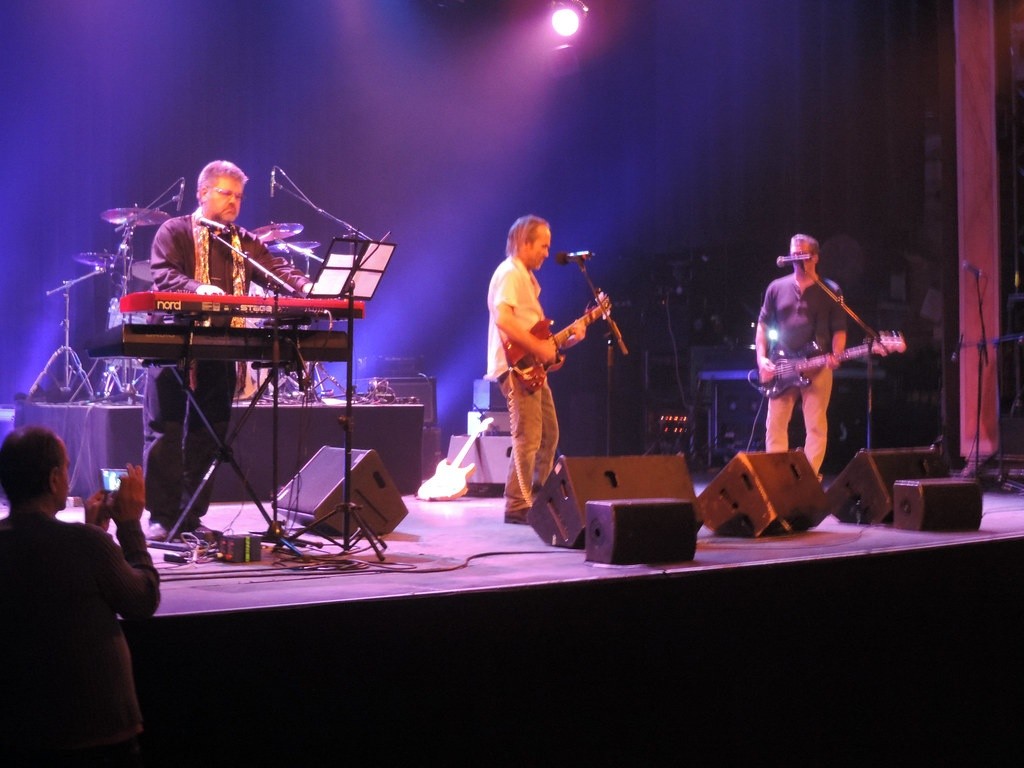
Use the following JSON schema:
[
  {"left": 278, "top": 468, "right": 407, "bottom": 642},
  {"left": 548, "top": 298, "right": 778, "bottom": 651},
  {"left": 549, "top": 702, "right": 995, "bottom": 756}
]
[
  {"left": 488, "top": 216, "right": 586, "bottom": 526},
  {"left": 756, "top": 235, "right": 847, "bottom": 484},
  {"left": 144, "top": 161, "right": 315, "bottom": 545},
  {"left": 0, "top": 427, "right": 160, "bottom": 768}
]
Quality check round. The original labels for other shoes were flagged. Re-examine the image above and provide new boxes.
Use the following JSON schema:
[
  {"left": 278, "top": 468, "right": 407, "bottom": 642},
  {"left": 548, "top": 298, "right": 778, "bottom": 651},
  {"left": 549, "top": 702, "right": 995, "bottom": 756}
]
[
  {"left": 145, "top": 519, "right": 168, "bottom": 542},
  {"left": 185, "top": 525, "right": 223, "bottom": 541},
  {"left": 505, "top": 507, "right": 531, "bottom": 525}
]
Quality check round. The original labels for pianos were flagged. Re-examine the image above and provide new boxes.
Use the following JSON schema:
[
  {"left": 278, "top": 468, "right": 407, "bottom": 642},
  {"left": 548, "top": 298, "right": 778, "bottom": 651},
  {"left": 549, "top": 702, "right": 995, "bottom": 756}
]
[
  {"left": 83, "top": 286, "right": 366, "bottom": 552},
  {"left": 689, "top": 342, "right": 891, "bottom": 467}
]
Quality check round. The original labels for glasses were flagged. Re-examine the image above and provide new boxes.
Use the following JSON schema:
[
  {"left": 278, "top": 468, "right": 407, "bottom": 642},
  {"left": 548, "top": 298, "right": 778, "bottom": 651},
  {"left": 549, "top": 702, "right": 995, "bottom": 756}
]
[{"left": 207, "top": 186, "right": 243, "bottom": 200}]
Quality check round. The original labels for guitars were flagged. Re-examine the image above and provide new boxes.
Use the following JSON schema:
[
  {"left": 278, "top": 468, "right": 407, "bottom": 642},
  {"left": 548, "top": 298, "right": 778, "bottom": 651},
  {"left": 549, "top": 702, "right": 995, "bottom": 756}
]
[
  {"left": 502, "top": 292, "right": 612, "bottom": 396},
  {"left": 417, "top": 416, "right": 495, "bottom": 501},
  {"left": 755, "top": 329, "right": 908, "bottom": 400}
]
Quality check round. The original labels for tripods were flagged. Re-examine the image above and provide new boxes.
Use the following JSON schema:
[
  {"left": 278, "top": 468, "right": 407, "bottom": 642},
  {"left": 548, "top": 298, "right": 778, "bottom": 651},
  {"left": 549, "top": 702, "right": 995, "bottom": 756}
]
[
  {"left": 27, "top": 219, "right": 148, "bottom": 404},
  {"left": 272, "top": 180, "right": 388, "bottom": 561},
  {"left": 207, "top": 226, "right": 324, "bottom": 564}
]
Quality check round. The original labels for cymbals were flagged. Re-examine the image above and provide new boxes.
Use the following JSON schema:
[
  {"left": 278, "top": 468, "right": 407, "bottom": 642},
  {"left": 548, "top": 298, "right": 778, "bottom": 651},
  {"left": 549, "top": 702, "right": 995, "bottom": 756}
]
[
  {"left": 70, "top": 251, "right": 134, "bottom": 269},
  {"left": 130, "top": 259, "right": 155, "bottom": 284},
  {"left": 252, "top": 222, "right": 304, "bottom": 243},
  {"left": 270, "top": 241, "right": 321, "bottom": 254},
  {"left": 100, "top": 207, "right": 172, "bottom": 226}
]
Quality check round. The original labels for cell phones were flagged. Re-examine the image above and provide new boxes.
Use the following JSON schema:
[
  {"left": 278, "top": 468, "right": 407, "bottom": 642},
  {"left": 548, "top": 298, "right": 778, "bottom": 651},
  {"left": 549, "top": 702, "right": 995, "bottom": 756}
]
[{"left": 100, "top": 468, "right": 129, "bottom": 492}]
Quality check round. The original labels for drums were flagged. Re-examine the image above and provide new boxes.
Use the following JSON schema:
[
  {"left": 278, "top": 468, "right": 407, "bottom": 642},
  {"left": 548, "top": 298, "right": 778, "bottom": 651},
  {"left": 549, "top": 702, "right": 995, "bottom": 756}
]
[{"left": 107, "top": 296, "right": 148, "bottom": 331}]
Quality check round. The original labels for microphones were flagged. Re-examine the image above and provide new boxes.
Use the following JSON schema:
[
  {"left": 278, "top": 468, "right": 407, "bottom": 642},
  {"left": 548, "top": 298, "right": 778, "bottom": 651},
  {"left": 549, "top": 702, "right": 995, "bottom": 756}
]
[
  {"left": 556, "top": 250, "right": 595, "bottom": 266},
  {"left": 776, "top": 256, "right": 810, "bottom": 267},
  {"left": 177, "top": 180, "right": 185, "bottom": 211},
  {"left": 271, "top": 168, "right": 275, "bottom": 197},
  {"left": 962, "top": 261, "right": 983, "bottom": 276},
  {"left": 196, "top": 217, "right": 230, "bottom": 233}
]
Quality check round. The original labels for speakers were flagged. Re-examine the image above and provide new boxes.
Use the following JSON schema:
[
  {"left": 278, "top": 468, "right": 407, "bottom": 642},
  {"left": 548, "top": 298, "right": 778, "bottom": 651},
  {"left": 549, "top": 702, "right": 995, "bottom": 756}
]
[
  {"left": 447, "top": 379, "right": 516, "bottom": 497},
  {"left": 696, "top": 449, "right": 830, "bottom": 537},
  {"left": 352, "top": 377, "right": 438, "bottom": 427},
  {"left": 272, "top": 445, "right": 409, "bottom": 537},
  {"left": 526, "top": 452, "right": 706, "bottom": 549},
  {"left": 893, "top": 478, "right": 983, "bottom": 533},
  {"left": 819, "top": 446, "right": 949, "bottom": 526},
  {"left": 583, "top": 500, "right": 696, "bottom": 565},
  {"left": 420, "top": 426, "right": 441, "bottom": 481}
]
